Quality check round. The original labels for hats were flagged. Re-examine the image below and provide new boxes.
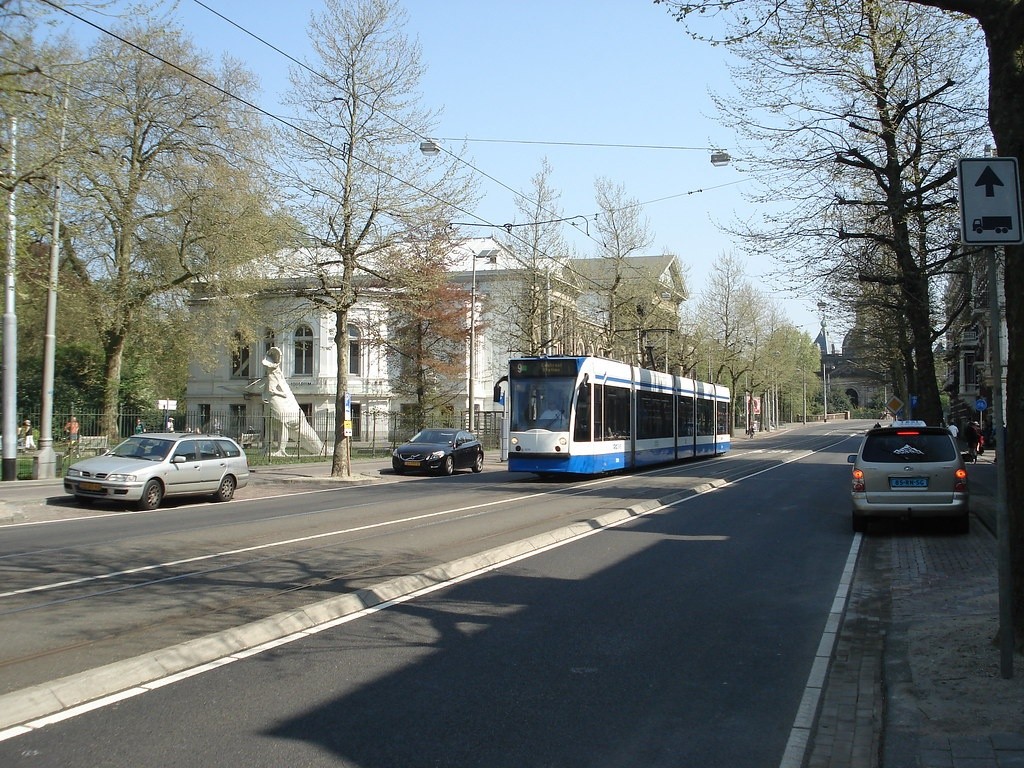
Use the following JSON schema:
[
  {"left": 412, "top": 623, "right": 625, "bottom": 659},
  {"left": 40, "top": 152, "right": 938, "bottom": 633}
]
[
  {"left": 974, "top": 421, "right": 980, "bottom": 426},
  {"left": 24, "top": 420, "right": 30, "bottom": 423}
]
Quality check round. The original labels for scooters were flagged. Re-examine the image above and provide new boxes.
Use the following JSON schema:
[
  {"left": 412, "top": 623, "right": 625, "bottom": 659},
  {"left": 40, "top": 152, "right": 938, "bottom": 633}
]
[{"left": 749, "top": 430, "right": 755, "bottom": 440}]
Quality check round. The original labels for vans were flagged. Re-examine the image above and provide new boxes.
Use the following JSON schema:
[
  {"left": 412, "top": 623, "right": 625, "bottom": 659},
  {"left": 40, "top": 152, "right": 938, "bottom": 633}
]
[{"left": 847, "top": 425, "right": 974, "bottom": 536}]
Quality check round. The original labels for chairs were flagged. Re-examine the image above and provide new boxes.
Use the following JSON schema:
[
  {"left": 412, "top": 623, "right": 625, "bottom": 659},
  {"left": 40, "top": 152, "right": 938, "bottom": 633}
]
[
  {"left": 150, "top": 445, "right": 164, "bottom": 456},
  {"left": 181, "top": 445, "right": 195, "bottom": 458}
]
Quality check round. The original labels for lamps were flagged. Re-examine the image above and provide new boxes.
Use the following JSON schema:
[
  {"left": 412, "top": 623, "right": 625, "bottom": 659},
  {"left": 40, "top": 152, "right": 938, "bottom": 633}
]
[
  {"left": 419, "top": 142, "right": 441, "bottom": 156},
  {"left": 710, "top": 152, "right": 731, "bottom": 167}
]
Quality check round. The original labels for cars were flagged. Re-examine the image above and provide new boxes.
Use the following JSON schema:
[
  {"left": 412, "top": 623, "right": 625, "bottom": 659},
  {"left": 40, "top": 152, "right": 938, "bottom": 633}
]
[
  {"left": 951, "top": 422, "right": 982, "bottom": 465},
  {"left": 63, "top": 430, "right": 253, "bottom": 511},
  {"left": 392, "top": 428, "right": 485, "bottom": 477}
]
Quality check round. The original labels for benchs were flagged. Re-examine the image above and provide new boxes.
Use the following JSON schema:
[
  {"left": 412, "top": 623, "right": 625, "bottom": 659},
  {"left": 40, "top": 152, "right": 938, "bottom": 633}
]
[
  {"left": 241, "top": 432, "right": 261, "bottom": 449},
  {"left": 75, "top": 435, "right": 108, "bottom": 456}
]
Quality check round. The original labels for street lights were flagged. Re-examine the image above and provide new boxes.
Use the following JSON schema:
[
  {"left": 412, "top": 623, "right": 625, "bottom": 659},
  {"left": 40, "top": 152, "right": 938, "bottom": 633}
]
[{"left": 468, "top": 248, "right": 499, "bottom": 434}]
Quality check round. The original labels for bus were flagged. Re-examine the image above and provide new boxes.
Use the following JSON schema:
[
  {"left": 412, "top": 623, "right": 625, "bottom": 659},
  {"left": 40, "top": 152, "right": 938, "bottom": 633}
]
[{"left": 493, "top": 352, "right": 733, "bottom": 478}]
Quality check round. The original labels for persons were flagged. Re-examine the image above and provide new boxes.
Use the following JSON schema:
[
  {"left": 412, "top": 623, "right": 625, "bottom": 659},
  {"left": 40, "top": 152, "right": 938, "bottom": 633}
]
[
  {"left": 749, "top": 426, "right": 756, "bottom": 438},
  {"left": 16, "top": 427, "right": 25, "bottom": 453},
  {"left": 213, "top": 418, "right": 221, "bottom": 434},
  {"left": 949, "top": 422, "right": 960, "bottom": 439},
  {"left": 136, "top": 418, "right": 146, "bottom": 434},
  {"left": 991, "top": 421, "right": 1006, "bottom": 462},
  {"left": 22, "top": 420, "right": 36, "bottom": 450},
  {"left": 966, "top": 416, "right": 993, "bottom": 464},
  {"left": 166, "top": 418, "right": 175, "bottom": 433},
  {"left": 185, "top": 424, "right": 208, "bottom": 434},
  {"left": 874, "top": 423, "right": 881, "bottom": 428},
  {"left": 248, "top": 425, "right": 254, "bottom": 431},
  {"left": 540, "top": 399, "right": 566, "bottom": 426},
  {"left": 65, "top": 417, "right": 80, "bottom": 459}
]
